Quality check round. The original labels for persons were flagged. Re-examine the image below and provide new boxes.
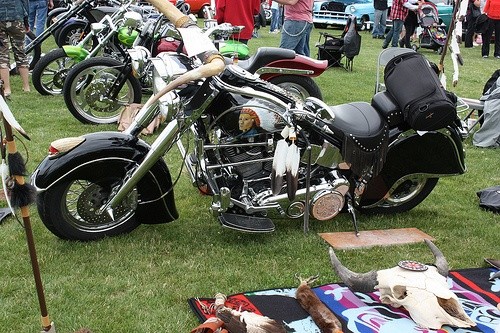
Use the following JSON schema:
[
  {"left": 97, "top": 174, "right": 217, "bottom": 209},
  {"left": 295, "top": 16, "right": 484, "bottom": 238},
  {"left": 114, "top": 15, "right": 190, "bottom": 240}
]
[
  {"left": 269, "top": 1, "right": 280, "bottom": 34},
  {"left": 274, "top": 0, "right": 313, "bottom": 57},
  {"left": 465, "top": 0, "right": 482, "bottom": 49},
  {"left": 25, "top": 0, "right": 53, "bottom": 46},
  {"left": 399, "top": 3, "right": 420, "bottom": 49},
  {"left": 481, "top": 0, "right": 500, "bottom": 59},
  {"left": 0, "top": 0, "right": 30, "bottom": 96},
  {"left": 381, "top": 0, "right": 422, "bottom": 49},
  {"left": 216, "top": 0, "right": 261, "bottom": 45},
  {"left": 372, "top": 0, "right": 388, "bottom": 39}
]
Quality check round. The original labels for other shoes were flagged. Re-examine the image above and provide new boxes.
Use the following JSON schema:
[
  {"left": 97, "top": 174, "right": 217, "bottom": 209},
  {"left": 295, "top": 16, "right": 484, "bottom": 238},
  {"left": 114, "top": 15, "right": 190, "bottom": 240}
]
[
  {"left": 483, "top": 55, "right": 488, "bottom": 58},
  {"left": 496, "top": 56, "right": 500, "bottom": 58},
  {"left": 270, "top": 29, "right": 280, "bottom": 34},
  {"left": 378, "top": 35, "right": 385, "bottom": 39}
]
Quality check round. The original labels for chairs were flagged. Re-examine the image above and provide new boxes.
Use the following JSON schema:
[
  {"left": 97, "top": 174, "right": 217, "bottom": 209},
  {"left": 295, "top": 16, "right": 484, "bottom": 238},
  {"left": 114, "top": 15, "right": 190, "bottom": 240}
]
[
  {"left": 314, "top": 13, "right": 362, "bottom": 73},
  {"left": 454, "top": 68, "right": 500, "bottom": 149}
]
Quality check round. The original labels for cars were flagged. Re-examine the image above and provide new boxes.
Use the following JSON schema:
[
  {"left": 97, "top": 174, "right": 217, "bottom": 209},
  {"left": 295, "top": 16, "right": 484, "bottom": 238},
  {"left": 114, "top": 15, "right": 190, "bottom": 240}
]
[
  {"left": 23, "top": 0, "right": 470, "bottom": 241},
  {"left": 312, "top": 0, "right": 373, "bottom": 29},
  {"left": 167, "top": 0, "right": 211, "bottom": 19},
  {"left": 344, "top": 0, "right": 454, "bottom": 38}
]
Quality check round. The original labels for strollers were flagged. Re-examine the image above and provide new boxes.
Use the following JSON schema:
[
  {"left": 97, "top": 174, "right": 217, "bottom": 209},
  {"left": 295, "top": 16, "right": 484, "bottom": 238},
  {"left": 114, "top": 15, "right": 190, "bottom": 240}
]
[{"left": 412, "top": 1, "right": 448, "bottom": 54}]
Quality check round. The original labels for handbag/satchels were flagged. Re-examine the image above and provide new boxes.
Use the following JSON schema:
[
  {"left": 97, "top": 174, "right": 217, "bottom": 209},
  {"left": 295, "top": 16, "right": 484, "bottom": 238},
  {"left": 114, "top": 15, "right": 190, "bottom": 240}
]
[{"left": 383, "top": 54, "right": 456, "bottom": 132}]
